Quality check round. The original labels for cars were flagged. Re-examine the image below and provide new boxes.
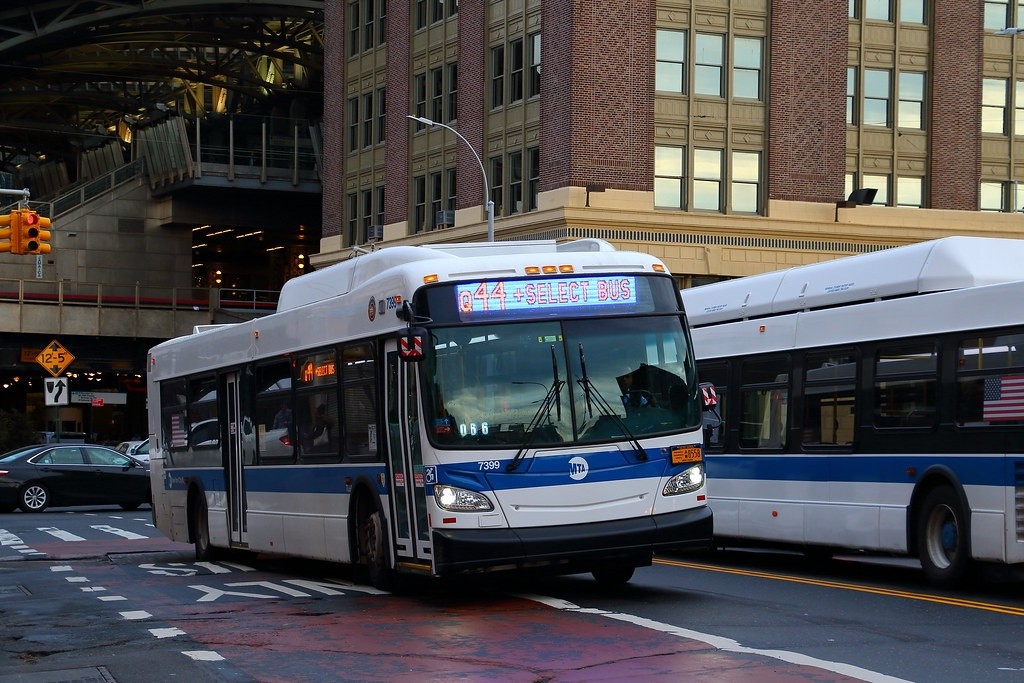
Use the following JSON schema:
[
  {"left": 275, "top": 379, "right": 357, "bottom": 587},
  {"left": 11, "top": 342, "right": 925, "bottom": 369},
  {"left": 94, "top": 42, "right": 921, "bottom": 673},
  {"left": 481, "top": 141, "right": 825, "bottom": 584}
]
[
  {"left": 0, "top": 439, "right": 151, "bottom": 512},
  {"left": 187, "top": 417, "right": 294, "bottom": 459}
]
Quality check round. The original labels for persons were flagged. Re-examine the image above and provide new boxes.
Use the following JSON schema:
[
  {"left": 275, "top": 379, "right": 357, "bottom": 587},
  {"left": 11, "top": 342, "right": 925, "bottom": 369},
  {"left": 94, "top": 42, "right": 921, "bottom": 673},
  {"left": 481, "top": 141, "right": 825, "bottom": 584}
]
[
  {"left": 96, "top": 431, "right": 149, "bottom": 442},
  {"left": 272, "top": 397, "right": 331, "bottom": 454},
  {"left": 585, "top": 364, "right": 653, "bottom": 428}
]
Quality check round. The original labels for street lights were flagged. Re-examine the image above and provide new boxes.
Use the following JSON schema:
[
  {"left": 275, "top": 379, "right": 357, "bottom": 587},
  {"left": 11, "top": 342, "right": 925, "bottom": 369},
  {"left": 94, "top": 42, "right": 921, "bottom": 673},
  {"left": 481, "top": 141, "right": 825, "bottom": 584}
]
[{"left": 403, "top": 112, "right": 497, "bottom": 241}]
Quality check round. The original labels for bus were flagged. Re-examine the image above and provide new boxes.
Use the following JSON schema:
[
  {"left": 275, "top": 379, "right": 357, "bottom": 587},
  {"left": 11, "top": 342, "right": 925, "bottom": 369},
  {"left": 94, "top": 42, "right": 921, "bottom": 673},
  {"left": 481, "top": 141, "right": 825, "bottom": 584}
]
[
  {"left": 642, "top": 233, "right": 1024, "bottom": 591},
  {"left": 145, "top": 236, "right": 728, "bottom": 600}
]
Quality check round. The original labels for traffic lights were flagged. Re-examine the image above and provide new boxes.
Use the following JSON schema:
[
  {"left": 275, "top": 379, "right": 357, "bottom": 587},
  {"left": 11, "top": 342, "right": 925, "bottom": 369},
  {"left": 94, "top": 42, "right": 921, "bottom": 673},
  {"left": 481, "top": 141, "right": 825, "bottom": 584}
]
[
  {"left": 36, "top": 214, "right": 54, "bottom": 254},
  {"left": 0, "top": 211, "right": 21, "bottom": 253},
  {"left": 16, "top": 210, "right": 43, "bottom": 255}
]
